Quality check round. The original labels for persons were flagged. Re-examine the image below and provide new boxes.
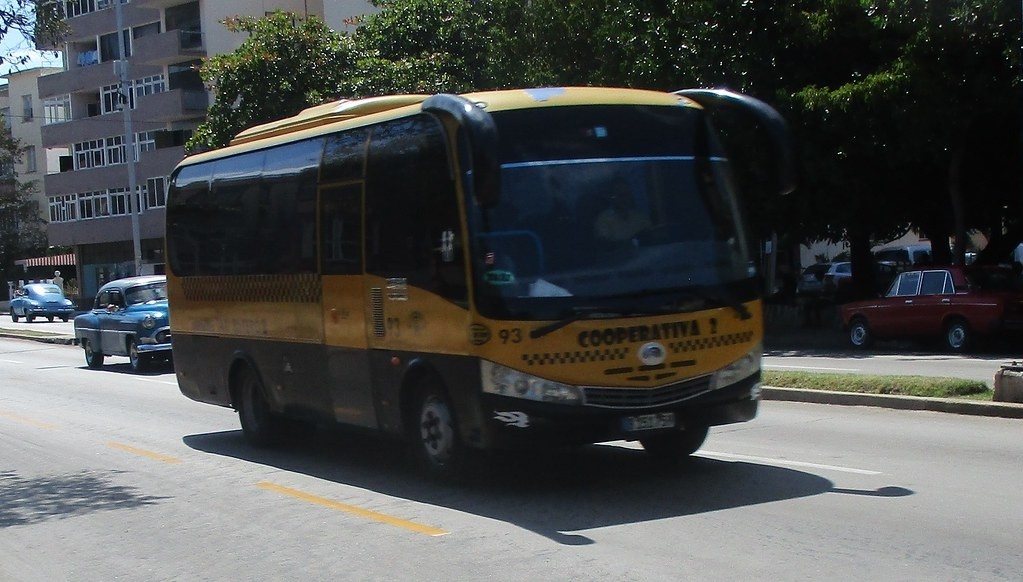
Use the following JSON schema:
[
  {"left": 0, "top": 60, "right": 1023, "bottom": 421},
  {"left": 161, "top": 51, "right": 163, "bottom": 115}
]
[
  {"left": 593, "top": 183, "right": 670, "bottom": 250},
  {"left": 182, "top": 177, "right": 323, "bottom": 269},
  {"left": 801, "top": 271, "right": 832, "bottom": 328}
]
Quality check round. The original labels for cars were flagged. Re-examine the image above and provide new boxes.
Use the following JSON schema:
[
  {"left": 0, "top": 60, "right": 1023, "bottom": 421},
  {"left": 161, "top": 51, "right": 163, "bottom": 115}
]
[
  {"left": 73, "top": 274, "right": 175, "bottom": 375},
  {"left": 823, "top": 263, "right": 899, "bottom": 303},
  {"left": 797, "top": 261, "right": 832, "bottom": 295},
  {"left": 950, "top": 243, "right": 982, "bottom": 267},
  {"left": 999, "top": 242, "right": 1022, "bottom": 271},
  {"left": 9, "top": 283, "right": 75, "bottom": 323},
  {"left": 875, "top": 245, "right": 932, "bottom": 274},
  {"left": 838, "top": 267, "right": 1023, "bottom": 354}
]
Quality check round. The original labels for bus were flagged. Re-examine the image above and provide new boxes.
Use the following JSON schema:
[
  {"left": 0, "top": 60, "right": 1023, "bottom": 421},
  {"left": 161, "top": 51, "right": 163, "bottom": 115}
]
[{"left": 164, "top": 88, "right": 787, "bottom": 484}]
